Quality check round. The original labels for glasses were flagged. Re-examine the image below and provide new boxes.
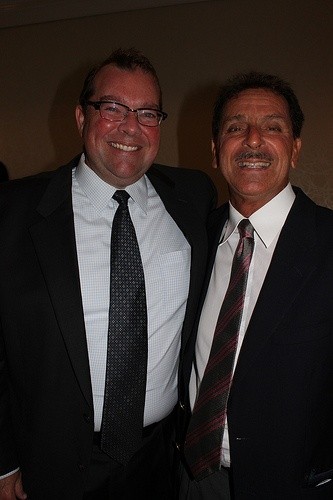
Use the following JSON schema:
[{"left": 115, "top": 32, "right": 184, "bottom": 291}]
[{"left": 84, "top": 100, "right": 168, "bottom": 128}]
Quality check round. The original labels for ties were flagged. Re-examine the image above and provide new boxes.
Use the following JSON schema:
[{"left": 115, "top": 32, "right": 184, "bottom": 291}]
[
  {"left": 183, "top": 219, "right": 256, "bottom": 483},
  {"left": 99, "top": 190, "right": 150, "bottom": 468}
]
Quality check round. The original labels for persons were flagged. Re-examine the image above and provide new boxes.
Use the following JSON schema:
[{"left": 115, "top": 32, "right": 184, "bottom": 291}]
[
  {"left": 2, "top": 48, "right": 216, "bottom": 500},
  {"left": 181, "top": 75, "right": 333, "bottom": 499}
]
